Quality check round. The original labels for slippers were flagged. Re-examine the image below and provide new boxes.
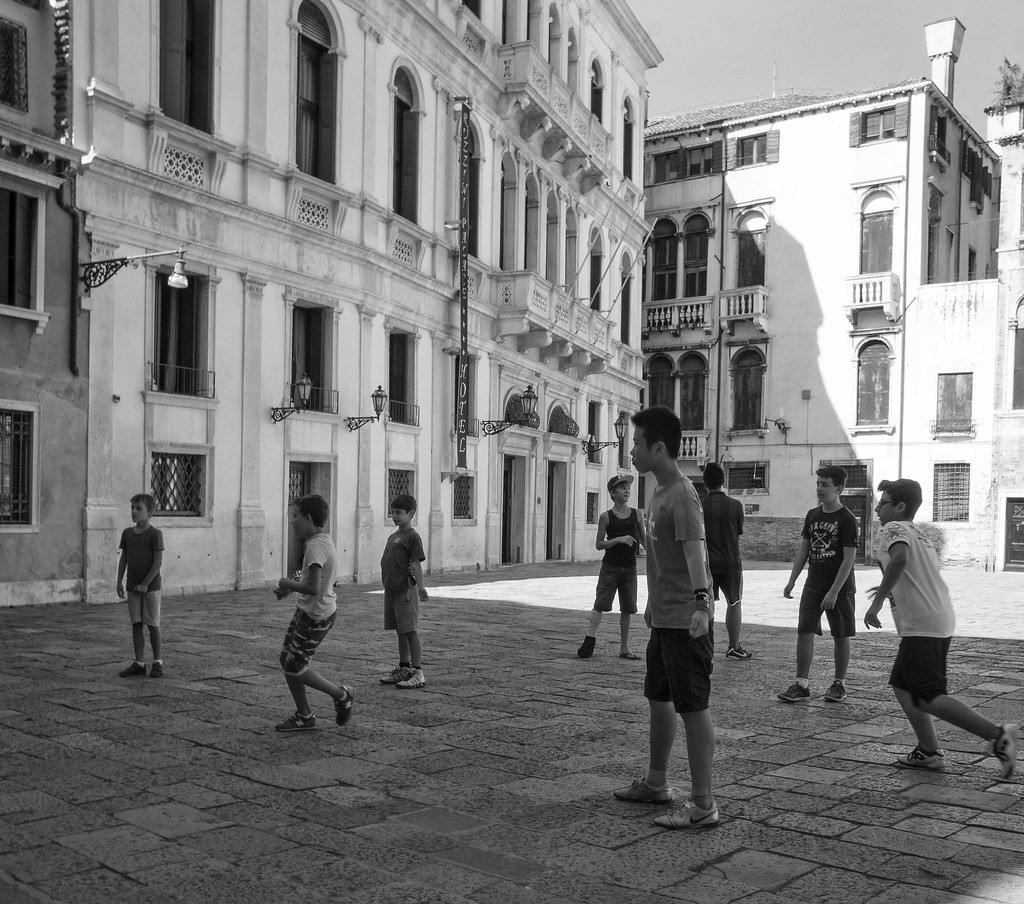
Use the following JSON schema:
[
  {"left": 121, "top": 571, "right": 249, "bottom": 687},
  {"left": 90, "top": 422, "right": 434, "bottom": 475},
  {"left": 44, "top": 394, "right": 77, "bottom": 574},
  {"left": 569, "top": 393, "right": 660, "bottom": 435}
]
[{"left": 619, "top": 652, "right": 641, "bottom": 659}]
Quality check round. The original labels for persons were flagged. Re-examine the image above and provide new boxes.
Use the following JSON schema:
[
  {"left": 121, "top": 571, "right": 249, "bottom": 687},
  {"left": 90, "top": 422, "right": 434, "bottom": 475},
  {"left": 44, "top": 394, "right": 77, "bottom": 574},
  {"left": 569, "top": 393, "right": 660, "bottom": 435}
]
[
  {"left": 578, "top": 475, "right": 645, "bottom": 661},
  {"left": 272, "top": 494, "right": 357, "bottom": 732},
  {"left": 777, "top": 466, "right": 859, "bottom": 702},
  {"left": 116, "top": 494, "right": 165, "bottom": 678},
  {"left": 864, "top": 479, "right": 1017, "bottom": 779},
  {"left": 700, "top": 463, "right": 752, "bottom": 660},
  {"left": 614, "top": 406, "right": 719, "bottom": 829},
  {"left": 379, "top": 494, "right": 429, "bottom": 690}
]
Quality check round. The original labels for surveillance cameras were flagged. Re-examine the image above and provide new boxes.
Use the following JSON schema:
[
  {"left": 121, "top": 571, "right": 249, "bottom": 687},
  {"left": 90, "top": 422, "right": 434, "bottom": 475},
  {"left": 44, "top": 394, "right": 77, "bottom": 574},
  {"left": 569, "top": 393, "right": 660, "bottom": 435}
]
[{"left": 584, "top": 434, "right": 591, "bottom": 443}]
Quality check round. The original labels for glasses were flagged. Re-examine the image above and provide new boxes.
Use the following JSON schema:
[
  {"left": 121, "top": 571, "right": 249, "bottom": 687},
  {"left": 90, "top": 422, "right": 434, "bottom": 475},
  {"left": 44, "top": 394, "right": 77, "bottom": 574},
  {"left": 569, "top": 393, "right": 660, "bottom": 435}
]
[{"left": 879, "top": 498, "right": 903, "bottom": 506}]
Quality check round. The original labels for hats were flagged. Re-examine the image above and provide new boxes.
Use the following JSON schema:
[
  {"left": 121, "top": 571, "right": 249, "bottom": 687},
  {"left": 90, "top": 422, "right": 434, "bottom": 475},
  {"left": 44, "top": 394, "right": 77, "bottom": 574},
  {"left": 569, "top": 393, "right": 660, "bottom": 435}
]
[{"left": 607, "top": 474, "right": 634, "bottom": 491}]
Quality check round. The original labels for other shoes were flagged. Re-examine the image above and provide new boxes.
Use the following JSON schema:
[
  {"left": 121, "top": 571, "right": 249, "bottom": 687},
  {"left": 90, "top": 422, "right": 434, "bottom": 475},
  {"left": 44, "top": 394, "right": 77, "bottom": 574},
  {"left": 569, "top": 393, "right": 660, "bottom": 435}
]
[
  {"left": 577, "top": 636, "right": 596, "bottom": 658},
  {"left": 120, "top": 662, "right": 147, "bottom": 677},
  {"left": 150, "top": 662, "right": 164, "bottom": 677}
]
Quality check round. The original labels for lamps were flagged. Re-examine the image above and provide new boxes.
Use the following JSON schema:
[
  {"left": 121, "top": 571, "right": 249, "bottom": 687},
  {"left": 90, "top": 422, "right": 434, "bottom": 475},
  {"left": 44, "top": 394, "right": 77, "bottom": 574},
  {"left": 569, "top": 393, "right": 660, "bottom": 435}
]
[
  {"left": 79, "top": 244, "right": 190, "bottom": 294},
  {"left": 762, "top": 417, "right": 787, "bottom": 433},
  {"left": 480, "top": 384, "right": 539, "bottom": 437},
  {"left": 582, "top": 412, "right": 629, "bottom": 455},
  {"left": 344, "top": 384, "right": 388, "bottom": 432},
  {"left": 271, "top": 372, "right": 313, "bottom": 424}
]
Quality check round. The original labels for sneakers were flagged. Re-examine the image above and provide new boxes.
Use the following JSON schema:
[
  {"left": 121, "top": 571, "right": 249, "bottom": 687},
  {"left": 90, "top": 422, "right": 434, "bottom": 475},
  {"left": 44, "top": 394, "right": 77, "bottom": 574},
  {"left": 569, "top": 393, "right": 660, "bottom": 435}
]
[
  {"left": 396, "top": 668, "right": 426, "bottom": 688},
  {"left": 613, "top": 776, "right": 672, "bottom": 804},
  {"left": 826, "top": 681, "right": 846, "bottom": 700},
  {"left": 275, "top": 713, "right": 316, "bottom": 730},
  {"left": 896, "top": 745, "right": 947, "bottom": 769},
  {"left": 993, "top": 724, "right": 1017, "bottom": 780},
  {"left": 726, "top": 645, "right": 752, "bottom": 660},
  {"left": 335, "top": 686, "right": 355, "bottom": 725},
  {"left": 379, "top": 667, "right": 408, "bottom": 684},
  {"left": 654, "top": 801, "right": 719, "bottom": 828},
  {"left": 777, "top": 682, "right": 811, "bottom": 701}
]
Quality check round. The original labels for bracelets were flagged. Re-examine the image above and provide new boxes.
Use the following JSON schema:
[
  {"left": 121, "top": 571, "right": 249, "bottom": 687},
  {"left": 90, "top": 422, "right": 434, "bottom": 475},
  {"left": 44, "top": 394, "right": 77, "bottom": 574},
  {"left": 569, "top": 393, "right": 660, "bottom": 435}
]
[{"left": 694, "top": 588, "right": 712, "bottom": 615}]
